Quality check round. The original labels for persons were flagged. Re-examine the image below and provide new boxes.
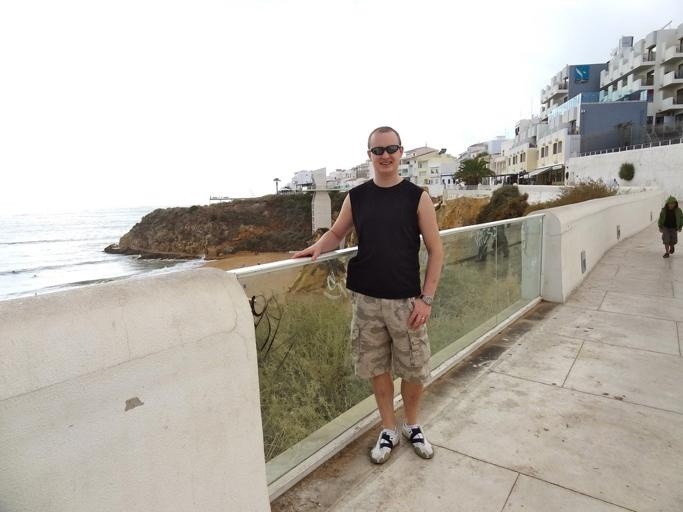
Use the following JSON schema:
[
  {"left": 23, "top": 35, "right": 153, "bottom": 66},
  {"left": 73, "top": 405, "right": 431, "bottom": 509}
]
[
  {"left": 285, "top": 126, "right": 445, "bottom": 464},
  {"left": 655, "top": 194, "right": 682, "bottom": 260}
]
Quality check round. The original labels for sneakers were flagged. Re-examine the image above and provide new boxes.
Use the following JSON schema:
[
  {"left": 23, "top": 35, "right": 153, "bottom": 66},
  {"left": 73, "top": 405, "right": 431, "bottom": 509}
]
[
  {"left": 402, "top": 423, "right": 434, "bottom": 459},
  {"left": 663, "top": 248, "right": 674, "bottom": 257},
  {"left": 370, "top": 428, "right": 400, "bottom": 464}
]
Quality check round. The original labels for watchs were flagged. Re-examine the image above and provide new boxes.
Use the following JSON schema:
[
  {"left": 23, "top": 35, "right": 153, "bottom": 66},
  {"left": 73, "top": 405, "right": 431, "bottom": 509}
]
[{"left": 417, "top": 293, "right": 434, "bottom": 306}]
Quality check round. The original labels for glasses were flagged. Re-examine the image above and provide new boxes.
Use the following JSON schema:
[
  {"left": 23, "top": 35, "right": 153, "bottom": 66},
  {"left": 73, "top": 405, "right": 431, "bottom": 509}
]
[{"left": 371, "top": 145, "right": 400, "bottom": 155}]
